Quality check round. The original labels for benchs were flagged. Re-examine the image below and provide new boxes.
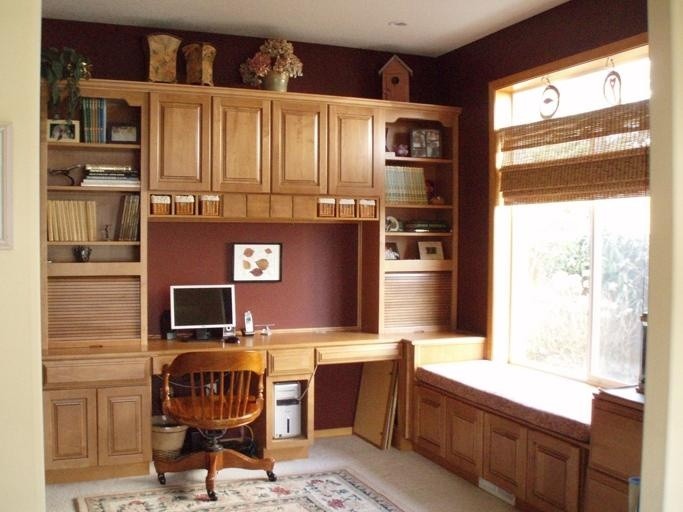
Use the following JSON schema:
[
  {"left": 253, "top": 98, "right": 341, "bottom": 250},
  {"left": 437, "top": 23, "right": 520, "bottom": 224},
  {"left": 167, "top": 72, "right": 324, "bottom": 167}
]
[{"left": 411, "top": 359, "right": 606, "bottom": 512}]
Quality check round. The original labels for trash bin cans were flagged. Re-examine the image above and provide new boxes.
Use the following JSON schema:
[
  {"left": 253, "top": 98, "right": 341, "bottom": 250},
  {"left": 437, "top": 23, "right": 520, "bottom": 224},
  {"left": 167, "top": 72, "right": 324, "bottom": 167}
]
[{"left": 151, "top": 414, "right": 189, "bottom": 462}]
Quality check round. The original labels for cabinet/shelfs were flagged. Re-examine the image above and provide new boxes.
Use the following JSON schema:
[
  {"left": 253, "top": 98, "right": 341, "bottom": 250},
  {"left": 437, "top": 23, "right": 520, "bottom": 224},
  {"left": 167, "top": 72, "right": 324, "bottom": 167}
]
[
  {"left": 42, "top": 355, "right": 153, "bottom": 487},
  {"left": 148, "top": 82, "right": 382, "bottom": 222},
  {"left": 41, "top": 77, "right": 149, "bottom": 354},
  {"left": 358, "top": 100, "right": 460, "bottom": 336},
  {"left": 580, "top": 385, "right": 644, "bottom": 512}
]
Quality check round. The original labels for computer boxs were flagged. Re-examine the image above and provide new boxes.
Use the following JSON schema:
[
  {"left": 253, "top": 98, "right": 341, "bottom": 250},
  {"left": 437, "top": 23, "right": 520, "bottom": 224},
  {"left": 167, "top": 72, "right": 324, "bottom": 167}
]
[{"left": 273, "top": 381, "right": 301, "bottom": 438}]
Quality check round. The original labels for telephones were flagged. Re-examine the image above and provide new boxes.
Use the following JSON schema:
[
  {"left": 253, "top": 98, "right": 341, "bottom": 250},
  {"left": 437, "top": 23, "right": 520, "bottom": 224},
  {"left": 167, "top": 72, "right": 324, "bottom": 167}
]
[{"left": 242, "top": 311, "right": 255, "bottom": 336}]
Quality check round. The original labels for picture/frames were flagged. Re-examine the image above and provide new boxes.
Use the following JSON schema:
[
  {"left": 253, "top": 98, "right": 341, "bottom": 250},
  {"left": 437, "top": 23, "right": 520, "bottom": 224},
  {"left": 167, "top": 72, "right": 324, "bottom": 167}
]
[
  {"left": 103, "top": 120, "right": 140, "bottom": 146},
  {"left": 232, "top": 242, "right": 282, "bottom": 282},
  {"left": 415, "top": 241, "right": 443, "bottom": 260},
  {"left": 45, "top": 118, "right": 80, "bottom": 143}
]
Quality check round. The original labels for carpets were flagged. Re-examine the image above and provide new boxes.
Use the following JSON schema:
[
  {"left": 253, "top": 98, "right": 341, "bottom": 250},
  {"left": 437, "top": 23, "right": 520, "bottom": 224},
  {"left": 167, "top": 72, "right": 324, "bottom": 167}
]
[{"left": 70, "top": 467, "right": 403, "bottom": 512}]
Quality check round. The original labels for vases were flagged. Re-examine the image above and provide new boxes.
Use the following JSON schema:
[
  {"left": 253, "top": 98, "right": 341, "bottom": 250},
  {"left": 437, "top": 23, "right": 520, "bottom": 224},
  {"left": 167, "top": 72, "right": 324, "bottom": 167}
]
[
  {"left": 260, "top": 71, "right": 288, "bottom": 91},
  {"left": 176, "top": 41, "right": 219, "bottom": 88},
  {"left": 138, "top": 30, "right": 184, "bottom": 85}
]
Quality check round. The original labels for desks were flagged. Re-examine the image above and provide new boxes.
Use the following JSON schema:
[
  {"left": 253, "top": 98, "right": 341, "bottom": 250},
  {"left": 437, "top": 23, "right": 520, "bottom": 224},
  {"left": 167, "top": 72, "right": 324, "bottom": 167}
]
[{"left": 150, "top": 327, "right": 485, "bottom": 464}]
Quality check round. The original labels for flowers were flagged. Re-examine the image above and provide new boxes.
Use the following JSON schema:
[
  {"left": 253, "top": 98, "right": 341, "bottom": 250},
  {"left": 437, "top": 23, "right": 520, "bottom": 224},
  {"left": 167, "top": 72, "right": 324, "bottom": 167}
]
[{"left": 238, "top": 37, "right": 303, "bottom": 86}]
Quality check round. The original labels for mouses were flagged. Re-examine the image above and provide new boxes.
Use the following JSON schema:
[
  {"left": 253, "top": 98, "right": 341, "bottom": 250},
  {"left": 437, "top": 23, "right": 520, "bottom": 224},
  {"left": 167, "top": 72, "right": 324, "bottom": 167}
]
[{"left": 221, "top": 335, "right": 241, "bottom": 343}]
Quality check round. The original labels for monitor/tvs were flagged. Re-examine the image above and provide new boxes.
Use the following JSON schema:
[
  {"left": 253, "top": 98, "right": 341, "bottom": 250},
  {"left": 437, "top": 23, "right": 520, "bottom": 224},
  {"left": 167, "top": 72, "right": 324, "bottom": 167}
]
[{"left": 167, "top": 282, "right": 237, "bottom": 338}]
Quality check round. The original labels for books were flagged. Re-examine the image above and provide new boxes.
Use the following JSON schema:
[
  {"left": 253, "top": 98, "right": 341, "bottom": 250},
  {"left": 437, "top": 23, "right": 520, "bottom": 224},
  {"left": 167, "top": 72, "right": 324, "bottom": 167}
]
[
  {"left": 116, "top": 193, "right": 139, "bottom": 241},
  {"left": 78, "top": 162, "right": 140, "bottom": 189},
  {"left": 404, "top": 221, "right": 449, "bottom": 229},
  {"left": 408, "top": 227, "right": 450, "bottom": 233},
  {"left": 384, "top": 166, "right": 429, "bottom": 206},
  {"left": 82, "top": 97, "right": 109, "bottom": 144},
  {"left": 46, "top": 198, "right": 98, "bottom": 243}
]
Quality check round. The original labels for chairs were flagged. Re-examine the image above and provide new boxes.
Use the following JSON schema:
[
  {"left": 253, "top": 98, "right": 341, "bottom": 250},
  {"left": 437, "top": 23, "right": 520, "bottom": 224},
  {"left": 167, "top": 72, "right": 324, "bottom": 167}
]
[{"left": 158, "top": 351, "right": 275, "bottom": 501}]
